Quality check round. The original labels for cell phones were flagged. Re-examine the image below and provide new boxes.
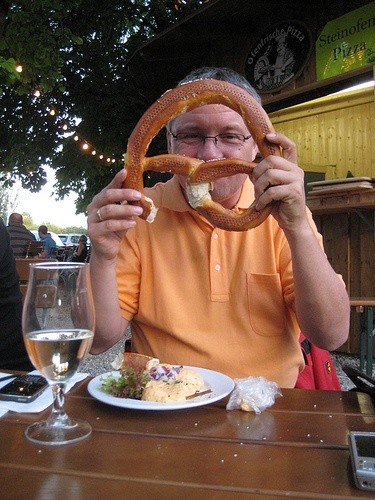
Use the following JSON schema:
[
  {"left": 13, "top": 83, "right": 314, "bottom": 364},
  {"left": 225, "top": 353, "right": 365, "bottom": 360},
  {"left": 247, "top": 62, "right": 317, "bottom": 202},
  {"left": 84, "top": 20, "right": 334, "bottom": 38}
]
[
  {"left": 0, "top": 375, "right": 49, "bottom": 402},
  {"left": 347, "top": 431, "right": 375, "bottom": 492}
]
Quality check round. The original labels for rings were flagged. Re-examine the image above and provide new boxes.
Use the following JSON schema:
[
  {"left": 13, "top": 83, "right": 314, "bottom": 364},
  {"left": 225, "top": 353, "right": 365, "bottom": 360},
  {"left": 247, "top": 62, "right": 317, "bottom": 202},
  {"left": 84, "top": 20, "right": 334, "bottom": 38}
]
[{"left": 97, "top": 209, "right": 106, "bottom": 221}]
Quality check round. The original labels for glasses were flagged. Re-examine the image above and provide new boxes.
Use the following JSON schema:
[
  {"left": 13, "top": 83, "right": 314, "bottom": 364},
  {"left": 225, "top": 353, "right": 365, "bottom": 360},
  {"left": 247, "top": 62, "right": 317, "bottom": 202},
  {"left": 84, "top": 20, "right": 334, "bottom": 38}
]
[{"left": 168, "top": 124, "right": 255, "bottom": 150}]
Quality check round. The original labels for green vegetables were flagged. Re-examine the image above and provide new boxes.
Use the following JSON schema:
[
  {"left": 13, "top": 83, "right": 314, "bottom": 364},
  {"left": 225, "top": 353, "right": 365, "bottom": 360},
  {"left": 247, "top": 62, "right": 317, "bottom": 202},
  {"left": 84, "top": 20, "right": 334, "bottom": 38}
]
[{"left": 99, "top": 367, "right": 180, "bottom": 399}]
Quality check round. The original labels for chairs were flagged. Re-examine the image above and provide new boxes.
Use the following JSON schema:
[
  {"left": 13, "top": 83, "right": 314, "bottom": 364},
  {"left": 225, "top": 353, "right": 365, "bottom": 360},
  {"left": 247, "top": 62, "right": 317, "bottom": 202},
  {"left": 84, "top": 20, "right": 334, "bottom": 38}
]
[{"left": 15, "top": 241, "right": 91, "bottom": 330}]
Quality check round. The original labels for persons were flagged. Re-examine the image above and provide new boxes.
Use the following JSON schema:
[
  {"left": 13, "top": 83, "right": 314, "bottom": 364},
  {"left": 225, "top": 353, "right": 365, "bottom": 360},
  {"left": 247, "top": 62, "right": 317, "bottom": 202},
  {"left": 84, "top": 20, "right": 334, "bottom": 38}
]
[
  {"left": 71, "top": 68, "right": 350, "bottom": 388},
  {"left": 0, "top": 218, "right": 36, "bottom": 370},
  {"left": 70, "top": 235, "right": 88, "bottom": 262},
  {"left": 38, "top": 225, "right": 56, "bottom": 257},
  {"left": 6, "top": 213, "right": 35, "bottom": 256}
]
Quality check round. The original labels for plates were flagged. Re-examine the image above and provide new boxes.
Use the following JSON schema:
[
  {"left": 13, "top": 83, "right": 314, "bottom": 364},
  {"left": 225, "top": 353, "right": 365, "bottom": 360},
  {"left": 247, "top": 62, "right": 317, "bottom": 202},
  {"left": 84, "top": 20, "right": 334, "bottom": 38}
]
[{"left": 86, "top": 365, "right": 236, "bottom": 411}]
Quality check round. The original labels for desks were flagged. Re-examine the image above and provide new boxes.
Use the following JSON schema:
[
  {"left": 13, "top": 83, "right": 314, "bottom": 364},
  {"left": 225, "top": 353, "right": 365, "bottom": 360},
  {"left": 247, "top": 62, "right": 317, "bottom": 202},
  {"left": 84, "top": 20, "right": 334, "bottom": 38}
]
[
  {"left": 349, "top": 297, "right": 375, "bottom": 377},
  {"left": 0, "top": 370, "right": 375, "bottom": 500}
]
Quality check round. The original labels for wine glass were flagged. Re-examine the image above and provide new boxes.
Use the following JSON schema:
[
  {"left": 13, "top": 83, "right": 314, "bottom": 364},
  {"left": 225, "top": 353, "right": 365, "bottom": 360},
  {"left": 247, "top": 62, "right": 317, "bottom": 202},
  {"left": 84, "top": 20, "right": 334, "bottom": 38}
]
[{"left": 21, "top": 262, "right": 96, "bottom": 446}]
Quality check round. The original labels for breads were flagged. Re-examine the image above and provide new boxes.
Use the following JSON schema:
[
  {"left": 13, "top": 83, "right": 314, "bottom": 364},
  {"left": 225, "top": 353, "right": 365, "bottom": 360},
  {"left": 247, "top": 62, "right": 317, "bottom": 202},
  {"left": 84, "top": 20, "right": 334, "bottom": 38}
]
[
  {"left": 119, "top": 352, "right": 160, "bottom": 373},
  {"left": 123, "top": 79, "right": 283, "bottom": 232}
]
